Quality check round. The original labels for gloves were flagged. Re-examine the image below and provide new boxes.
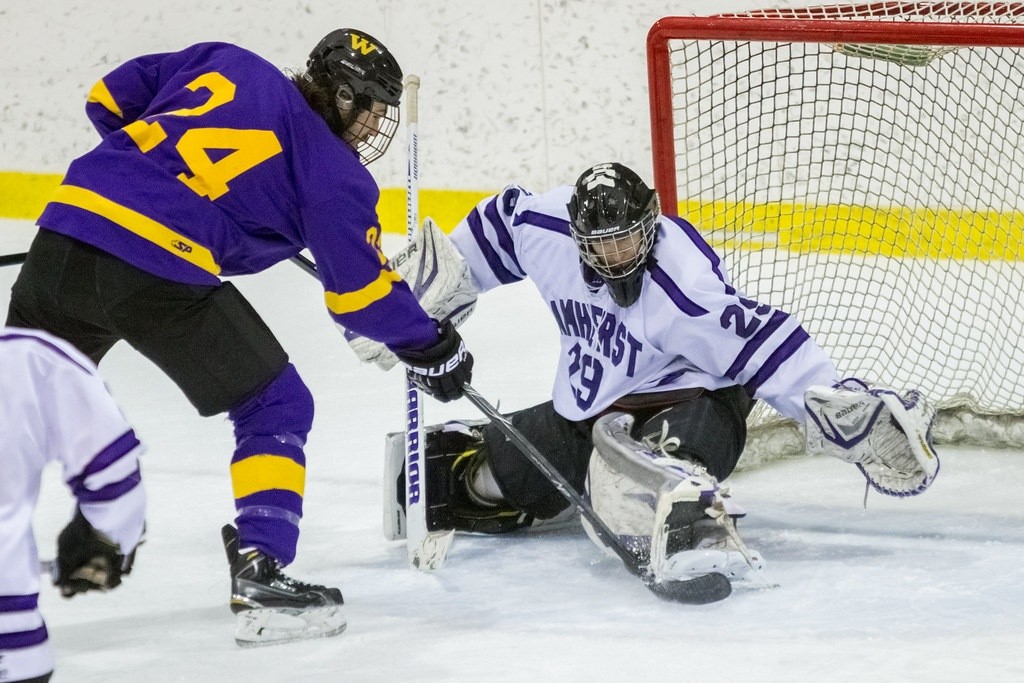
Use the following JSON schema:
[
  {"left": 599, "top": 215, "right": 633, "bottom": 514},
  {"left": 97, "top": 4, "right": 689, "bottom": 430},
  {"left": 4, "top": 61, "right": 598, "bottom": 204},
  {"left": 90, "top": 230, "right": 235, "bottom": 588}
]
[
  {"left": 395, "top": 318, "right": 473, "bottom": 403},
  {"left": 52, "top": 500, "right": 147, "bottom": 598}
]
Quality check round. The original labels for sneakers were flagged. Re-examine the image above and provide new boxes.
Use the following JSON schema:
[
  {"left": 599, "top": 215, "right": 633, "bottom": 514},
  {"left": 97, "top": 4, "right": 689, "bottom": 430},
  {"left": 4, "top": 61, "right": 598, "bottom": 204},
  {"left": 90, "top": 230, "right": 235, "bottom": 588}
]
[{"left": 222, "top": 525, "right": 347, "bottom": 642}]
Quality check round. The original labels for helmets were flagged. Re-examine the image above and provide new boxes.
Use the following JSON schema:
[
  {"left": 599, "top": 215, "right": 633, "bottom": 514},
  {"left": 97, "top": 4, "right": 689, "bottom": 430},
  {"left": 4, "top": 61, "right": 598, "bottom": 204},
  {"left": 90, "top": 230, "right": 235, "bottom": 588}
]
[
  {"left": 302, "top": 28, "right": 403, "bottom": 136},
  {"left": 566, "top": 162, "right": 662, "bottom": 308}
]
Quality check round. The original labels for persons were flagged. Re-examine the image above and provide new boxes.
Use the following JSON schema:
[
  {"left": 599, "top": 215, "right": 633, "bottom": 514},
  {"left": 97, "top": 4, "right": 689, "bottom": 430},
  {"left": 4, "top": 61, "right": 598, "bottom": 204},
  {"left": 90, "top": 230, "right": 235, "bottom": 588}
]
[
  {"left": 6, "top": 28, "right": 475, "bottom": 622},
  {"left": 0, "top": 327, "right": 146, "bottom": 683},
  {"left": 350, "top": 161, "right": 938, "bottom": 603}
]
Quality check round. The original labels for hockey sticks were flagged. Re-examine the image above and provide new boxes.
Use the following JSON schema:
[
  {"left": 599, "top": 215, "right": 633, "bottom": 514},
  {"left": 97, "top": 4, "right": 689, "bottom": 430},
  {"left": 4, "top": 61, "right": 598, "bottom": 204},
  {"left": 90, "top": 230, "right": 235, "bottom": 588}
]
[
  {"left": 290, "top": 251, "right": 734, "bottom": 606},
  {"left": 396, "top": 74, "right": 453, "bottom": 574}
]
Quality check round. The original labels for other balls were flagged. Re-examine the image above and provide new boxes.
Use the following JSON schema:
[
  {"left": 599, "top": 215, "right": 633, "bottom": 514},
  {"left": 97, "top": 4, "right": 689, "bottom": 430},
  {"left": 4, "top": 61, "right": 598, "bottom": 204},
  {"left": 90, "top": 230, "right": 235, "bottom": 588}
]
[{"left": 624, "top": 545, "right": 655, "bottom": 578}]
